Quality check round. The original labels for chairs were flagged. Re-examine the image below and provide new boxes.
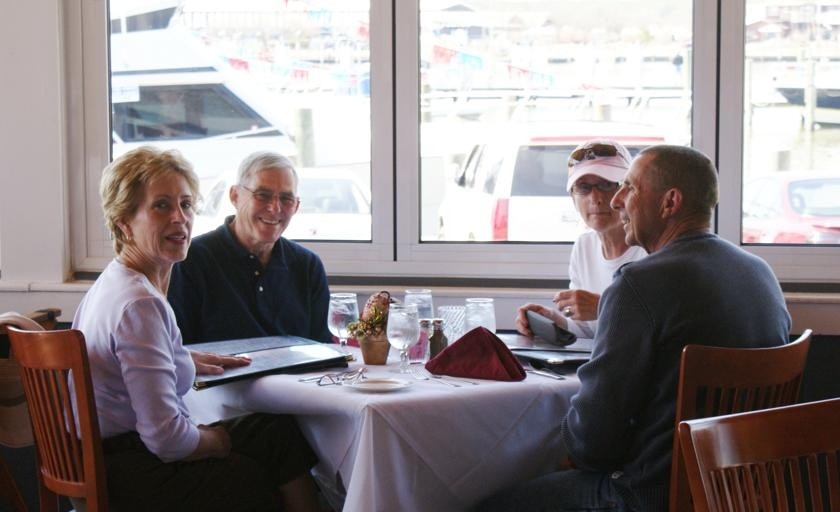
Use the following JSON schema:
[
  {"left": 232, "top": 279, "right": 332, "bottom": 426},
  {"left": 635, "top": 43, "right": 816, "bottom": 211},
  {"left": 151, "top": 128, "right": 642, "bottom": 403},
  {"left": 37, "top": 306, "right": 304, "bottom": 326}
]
[
  {"left": 669, "top": 328, "right": 813, "bottom": 512},
  {"left": 5, "top": 308, "right": 110, "bottom": 512},
  {"left": 678, "top": 397, "right": 839, "bottom": 511}
]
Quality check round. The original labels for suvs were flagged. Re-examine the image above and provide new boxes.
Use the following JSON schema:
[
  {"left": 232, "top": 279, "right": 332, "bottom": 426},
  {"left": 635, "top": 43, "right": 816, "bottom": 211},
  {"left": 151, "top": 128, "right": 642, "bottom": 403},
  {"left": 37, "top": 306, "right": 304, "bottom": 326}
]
[
  {"left": 434, "top": 133, "right": 665, "bottom": 246},
  {"left": 113, "top": 54, "right": 298, "bottom": 235}
]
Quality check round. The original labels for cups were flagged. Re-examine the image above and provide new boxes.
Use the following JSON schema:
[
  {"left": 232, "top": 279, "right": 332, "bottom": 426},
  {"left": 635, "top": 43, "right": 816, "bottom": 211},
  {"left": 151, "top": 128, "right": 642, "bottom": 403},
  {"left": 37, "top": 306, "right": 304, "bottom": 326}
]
[
  {"left": 405, "top": 289, "right": 433, "bottom": 320},
  {"left": 465, "top": 298, "right": 496, "bottom": 335},
  {"left": 437, "top": 305, "right": 464, "bottom": 346}
]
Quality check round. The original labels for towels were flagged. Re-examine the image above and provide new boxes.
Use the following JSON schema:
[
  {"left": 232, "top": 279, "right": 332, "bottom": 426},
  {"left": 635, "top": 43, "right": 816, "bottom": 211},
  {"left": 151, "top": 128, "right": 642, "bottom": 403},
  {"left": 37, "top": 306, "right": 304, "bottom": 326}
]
[{"left": 424, "top": 326, "right": 527, "bottom": 382}]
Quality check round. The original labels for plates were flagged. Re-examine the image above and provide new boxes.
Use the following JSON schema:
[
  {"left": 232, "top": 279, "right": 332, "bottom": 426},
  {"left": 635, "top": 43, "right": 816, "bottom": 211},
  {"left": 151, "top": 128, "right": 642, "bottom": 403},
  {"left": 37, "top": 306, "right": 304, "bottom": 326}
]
[{"left": 352, "top": 378, "right": 408, "bottom": 392}]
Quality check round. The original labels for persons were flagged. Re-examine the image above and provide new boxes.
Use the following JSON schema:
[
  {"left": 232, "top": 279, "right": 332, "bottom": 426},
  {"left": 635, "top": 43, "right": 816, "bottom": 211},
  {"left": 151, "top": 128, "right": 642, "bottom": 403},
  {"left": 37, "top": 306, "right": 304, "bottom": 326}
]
[
  {"left": 67, "top": 149, "right": 325, "bottom": 511},
  {"left": 469, "top": 144, "right": 792, "bottom": 510},
  {"left": 165, "top": 151, "right": 346, "bottom": 344},
  {"left": 514, "top": 141, "right": 650, "bottom": 344}
]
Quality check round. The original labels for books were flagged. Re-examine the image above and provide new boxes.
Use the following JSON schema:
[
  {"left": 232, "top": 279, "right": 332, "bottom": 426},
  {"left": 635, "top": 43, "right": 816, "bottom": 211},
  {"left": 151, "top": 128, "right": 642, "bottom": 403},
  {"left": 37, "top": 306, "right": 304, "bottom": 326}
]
[{"left": 175, "top": 328, "right": 357, "bottom": 392}]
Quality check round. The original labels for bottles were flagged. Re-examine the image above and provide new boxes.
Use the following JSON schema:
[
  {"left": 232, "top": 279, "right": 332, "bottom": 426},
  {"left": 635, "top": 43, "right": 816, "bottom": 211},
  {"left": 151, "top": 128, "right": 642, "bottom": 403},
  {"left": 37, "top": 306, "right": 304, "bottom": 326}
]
[{"left": 430, "top": 319, "right": 448, "bottom": 359}]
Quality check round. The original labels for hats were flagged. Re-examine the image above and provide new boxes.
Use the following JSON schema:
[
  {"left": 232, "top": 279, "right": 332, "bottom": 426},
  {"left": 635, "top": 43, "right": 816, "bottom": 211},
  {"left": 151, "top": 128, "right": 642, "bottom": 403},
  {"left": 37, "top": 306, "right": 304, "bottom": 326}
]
[{"left": 567, "top": 138, "right": 632, "bottom": 192}]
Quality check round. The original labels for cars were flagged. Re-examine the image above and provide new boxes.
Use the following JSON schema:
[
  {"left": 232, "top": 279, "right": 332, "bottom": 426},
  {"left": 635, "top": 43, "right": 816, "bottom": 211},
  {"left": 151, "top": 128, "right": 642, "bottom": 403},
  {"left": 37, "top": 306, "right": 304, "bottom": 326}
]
[{"left": 744, "top": 172, "right": 835, "bottom": 242}]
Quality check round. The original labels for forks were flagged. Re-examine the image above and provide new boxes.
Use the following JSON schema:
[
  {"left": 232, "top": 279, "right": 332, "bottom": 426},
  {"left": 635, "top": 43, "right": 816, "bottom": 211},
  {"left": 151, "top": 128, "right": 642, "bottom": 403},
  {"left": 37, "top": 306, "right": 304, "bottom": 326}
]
[{"left": 409, "top": 368, "right": 462, "bottom": 387}]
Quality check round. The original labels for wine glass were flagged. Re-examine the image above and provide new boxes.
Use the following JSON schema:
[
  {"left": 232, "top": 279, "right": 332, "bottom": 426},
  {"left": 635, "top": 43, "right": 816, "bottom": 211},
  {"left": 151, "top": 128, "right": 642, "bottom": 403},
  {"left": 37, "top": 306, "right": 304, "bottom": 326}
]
[
  {"left": 327, "top": 292, "right": 360, "bottom": 356},
  {"left": 386, "top": 303, "right": 420, "bottom": 373}
]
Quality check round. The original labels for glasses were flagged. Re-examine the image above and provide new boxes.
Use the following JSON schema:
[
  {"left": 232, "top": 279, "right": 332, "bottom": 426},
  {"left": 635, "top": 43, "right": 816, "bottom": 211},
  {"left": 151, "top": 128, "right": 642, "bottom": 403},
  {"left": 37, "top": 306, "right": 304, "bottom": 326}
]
[
  {"left": 568, "top": 144, "right": 629, "bottom": 167},
  {"left": 572, "top": 181, "right": 619, "bottom": 195},
  {"left": 300, "top": 368, "right": 364, "bottom": 387},
  {"left": 241, "top": 185, "right": 295, "bottom": 207}
]
